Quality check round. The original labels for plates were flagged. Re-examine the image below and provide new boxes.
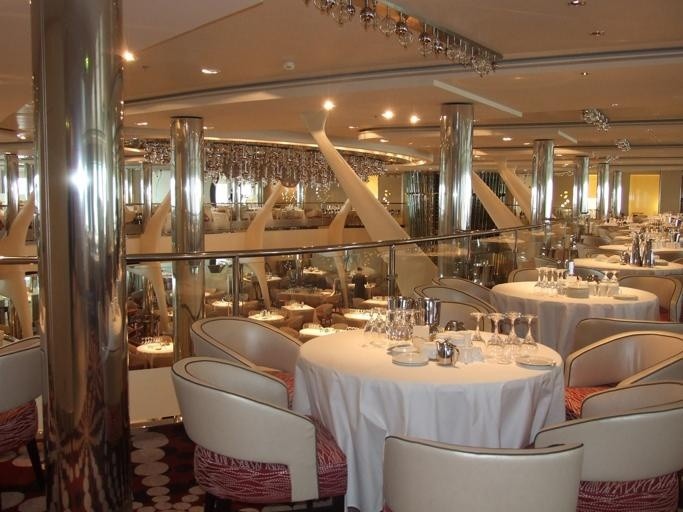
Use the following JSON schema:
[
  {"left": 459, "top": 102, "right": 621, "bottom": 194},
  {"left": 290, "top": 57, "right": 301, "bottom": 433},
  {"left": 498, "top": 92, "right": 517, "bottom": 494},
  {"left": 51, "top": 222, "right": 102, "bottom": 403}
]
[
  {"left": 389, "top": 345, "right": 430, "bottom": 366},
  {"left": 514, "top": 354, "right": 553, "bottom": 366}
]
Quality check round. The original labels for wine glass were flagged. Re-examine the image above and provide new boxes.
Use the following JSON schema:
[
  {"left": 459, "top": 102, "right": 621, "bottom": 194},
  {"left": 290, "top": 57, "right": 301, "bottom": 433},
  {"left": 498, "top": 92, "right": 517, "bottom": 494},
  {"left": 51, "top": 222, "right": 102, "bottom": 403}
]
[
  {"left": 486, "top": 313, "right": 505, "bottom": 358},
  {"left": 366, "top": 308, "right": 424, "bottom": 343},
  {"left": 520, "top": 314, "right": 539, "bottom": 357},
  {"left": 504, "top": 312, "right": 520, "bottom": 353},
  {"left": 625, "top": 214, "right": 670, "bottom": 248},
  {"left": 532, "top": 267, "right": 568, "bottom": 298},
  {"left": 470, "top": 312, "right": 486, "bottom": 354}
]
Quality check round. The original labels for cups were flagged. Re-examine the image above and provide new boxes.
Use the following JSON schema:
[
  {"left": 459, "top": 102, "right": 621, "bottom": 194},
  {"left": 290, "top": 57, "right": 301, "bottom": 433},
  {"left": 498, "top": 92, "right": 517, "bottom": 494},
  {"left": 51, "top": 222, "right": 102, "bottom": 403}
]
[{"left": 496, "top": 338, "right": 511, "bottom": 363}]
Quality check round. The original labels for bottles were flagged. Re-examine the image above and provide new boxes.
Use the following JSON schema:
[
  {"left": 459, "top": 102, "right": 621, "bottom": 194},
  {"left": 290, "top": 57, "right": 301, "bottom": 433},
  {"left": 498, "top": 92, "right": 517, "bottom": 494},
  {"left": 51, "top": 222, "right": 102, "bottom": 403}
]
[
  {"left": 388, "top": 297, "right": 441, "bottom": 326},
  {"left": 630, "top": 235, "right": 641, "bottom": 265},
  {"left": 669, "top": 213, "right": 681, "bottom": 242},
  {"left": 610, "top": 271, "right": 620, "bottom": 296},
  {"left": 619, "top": 250, "right": 628, "bottom": 264},
  {"left": 598, "top": 271, "right": 609, "bottom": 297},
  {"left": 642, "top": 237, "right": 654, "bottom": 267},
  {"left": 576, "top": 272, "right": 600, "bottom": 295}
]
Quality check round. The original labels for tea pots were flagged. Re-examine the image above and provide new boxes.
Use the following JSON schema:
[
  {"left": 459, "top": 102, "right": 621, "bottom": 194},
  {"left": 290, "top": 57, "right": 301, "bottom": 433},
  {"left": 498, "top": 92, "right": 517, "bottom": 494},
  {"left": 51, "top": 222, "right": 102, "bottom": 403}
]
[{"left": 437, "top": 339, "right": 459, "bottom": 365}]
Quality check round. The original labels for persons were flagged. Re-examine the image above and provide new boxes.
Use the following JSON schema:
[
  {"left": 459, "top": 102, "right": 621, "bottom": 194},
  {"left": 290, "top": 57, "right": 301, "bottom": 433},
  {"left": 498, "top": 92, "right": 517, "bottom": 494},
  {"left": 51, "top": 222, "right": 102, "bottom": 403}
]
[{"left": 351, "top": 267, "right": 367, "bottom": 300}]
